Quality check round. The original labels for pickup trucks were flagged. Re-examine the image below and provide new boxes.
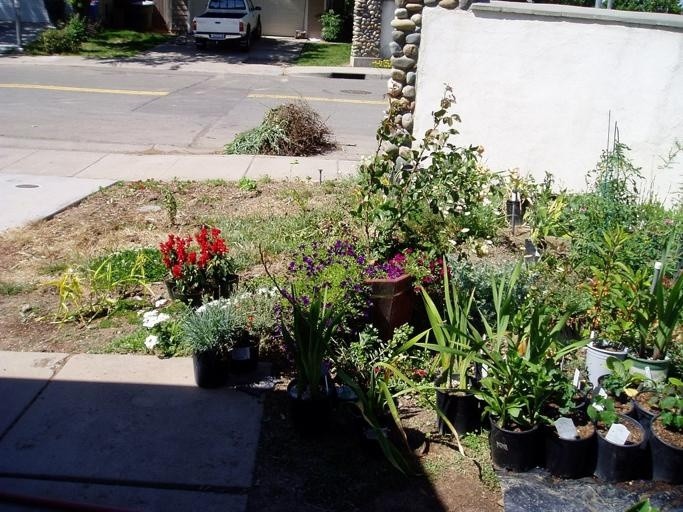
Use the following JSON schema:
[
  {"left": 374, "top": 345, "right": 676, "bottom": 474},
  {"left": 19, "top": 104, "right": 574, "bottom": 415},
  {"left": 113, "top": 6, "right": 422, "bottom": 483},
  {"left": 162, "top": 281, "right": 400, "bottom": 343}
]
[{"left": 192, "top": 1, "right": 262, "bottom": 48}]
